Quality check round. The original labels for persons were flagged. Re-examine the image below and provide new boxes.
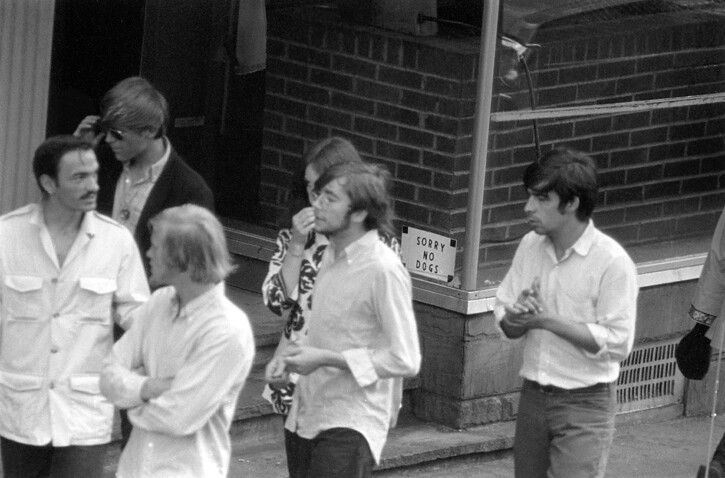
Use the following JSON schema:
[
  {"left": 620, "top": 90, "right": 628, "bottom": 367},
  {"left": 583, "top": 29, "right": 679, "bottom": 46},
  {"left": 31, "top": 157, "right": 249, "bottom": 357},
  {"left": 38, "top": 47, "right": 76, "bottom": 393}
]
[
  {"left": 494, "top": 150, "right": 639, "bottom": 478},
  {"left": 674, "top": 202, "right": 725, "bottom": 478},
  {"left": 74, "top": 76, "right": 214, "bottom": 280},
  {"left": 98, "top": 205, "right": 254, "bottom": 478},
  {"left": 261, "top": 136, "right": 421, "bottom": 478},
  {"left": 0, "top": 135, "right": 153, "bottom": 478}
]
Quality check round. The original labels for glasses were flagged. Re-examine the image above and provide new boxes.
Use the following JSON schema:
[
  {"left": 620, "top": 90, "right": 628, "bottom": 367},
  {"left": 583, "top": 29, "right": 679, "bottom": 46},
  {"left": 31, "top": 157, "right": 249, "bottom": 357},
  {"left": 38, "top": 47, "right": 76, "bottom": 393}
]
[
  {"left": 311, "top": 191, "right": 352, "bottom": 207},
  {"left": 95, "top": 122, "right": 131, "bottom": 141}
]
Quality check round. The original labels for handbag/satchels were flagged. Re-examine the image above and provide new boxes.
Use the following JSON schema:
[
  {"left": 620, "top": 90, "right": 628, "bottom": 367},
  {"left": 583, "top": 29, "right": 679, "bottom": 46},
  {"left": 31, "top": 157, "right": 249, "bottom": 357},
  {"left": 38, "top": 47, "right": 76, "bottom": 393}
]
[{"left": 675, "top": 321, "right": 713, "bottom": 381}]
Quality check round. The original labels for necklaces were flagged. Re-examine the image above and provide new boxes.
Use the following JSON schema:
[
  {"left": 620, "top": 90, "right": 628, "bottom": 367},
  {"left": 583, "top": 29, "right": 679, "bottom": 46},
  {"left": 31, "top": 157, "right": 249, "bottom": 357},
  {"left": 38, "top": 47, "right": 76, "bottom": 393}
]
[{"left": 117, "top": 166, "right": 148, "bottom": 221}]
